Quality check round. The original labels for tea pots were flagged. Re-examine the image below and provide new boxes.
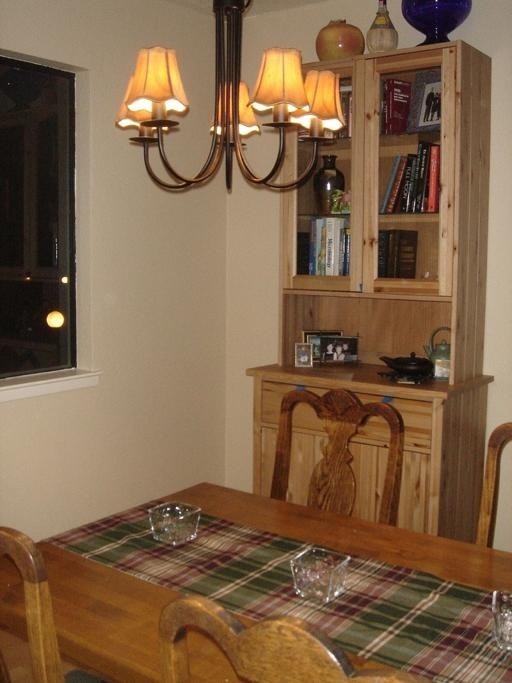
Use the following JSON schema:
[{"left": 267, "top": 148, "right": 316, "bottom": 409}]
[
  {"left": 423, "top": 324, "right": 451, "bottom": 381},
  {"left": 379, "top": 350, "right": 433, "bottom": 381}
]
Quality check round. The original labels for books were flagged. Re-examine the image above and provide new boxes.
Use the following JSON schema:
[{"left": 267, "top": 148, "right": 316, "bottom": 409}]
[
  {"left": 336, "top": 76, "right": 413, "bottom": 137},
  {"left": 295, "top": 217, "right": 419, "bottom": 280},
  {"left": 379, "top": 142, "right": 446, "bottom": 214}
]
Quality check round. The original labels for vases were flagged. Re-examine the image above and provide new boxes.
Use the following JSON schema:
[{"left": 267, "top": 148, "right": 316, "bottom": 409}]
[
  {"left": 315, "top": 20, "right": 364, "bottom": 61},
  {"left": 402, "top": 0, "right": 472, "bottom": 48}
]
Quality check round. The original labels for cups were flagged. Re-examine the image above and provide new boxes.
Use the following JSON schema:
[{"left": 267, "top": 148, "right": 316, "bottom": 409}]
[
  {"left": 147, "top": 498, "right": 202, "bottom": 548},
  {"left": 490, "top": 588, "right": 512, "bottom": 651},
  {"left": 290, "top": 545, "right": 352, "bottom": 605}
]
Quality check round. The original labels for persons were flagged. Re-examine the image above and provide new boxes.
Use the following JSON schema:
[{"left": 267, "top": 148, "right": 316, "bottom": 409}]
[
  {"left": 297, "top": 346, "right": 309, "bottom": 362},
  {"left": 321, "top": 341, "right": 352, "bottom": 362},
  {"left": 421, "top": 88, "right": 441, "bottom": 123}
]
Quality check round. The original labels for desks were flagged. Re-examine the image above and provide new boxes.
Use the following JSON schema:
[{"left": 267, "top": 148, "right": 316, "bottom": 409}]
[{"left": 1, "top": 481, "right": 512, "bottom": 681}]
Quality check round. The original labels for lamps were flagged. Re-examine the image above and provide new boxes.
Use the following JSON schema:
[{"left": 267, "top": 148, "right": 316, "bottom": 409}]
[{"left": 114, "top": 1, "right": 345, "bottom": 190}]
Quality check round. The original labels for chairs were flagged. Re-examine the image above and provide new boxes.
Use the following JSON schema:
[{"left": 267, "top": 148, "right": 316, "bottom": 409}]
[
  {"left": 159, "top": 597, "right": 425, "bottom": 682},
  {"left": 474, "top": 411, "right": 511, "bottom": 548},
  {"left": 0, "top": 526, "right": 106, "bottom": 683}
]
